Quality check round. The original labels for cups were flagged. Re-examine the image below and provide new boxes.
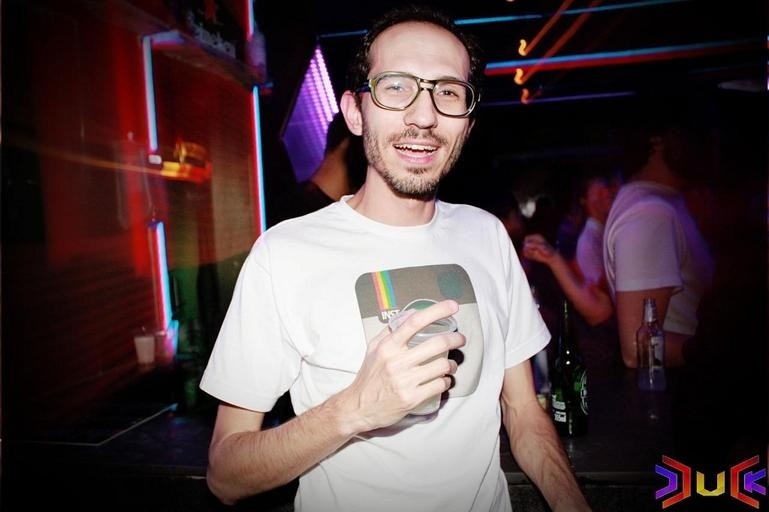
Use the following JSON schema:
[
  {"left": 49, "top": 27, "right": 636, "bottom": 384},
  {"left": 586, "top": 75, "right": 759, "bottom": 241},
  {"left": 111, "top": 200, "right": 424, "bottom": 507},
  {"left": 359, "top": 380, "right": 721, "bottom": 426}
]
[
  {"left": 133, "top": 336, "right": 156, "bottom": 364},
  {"left": 387, "top": 308, "right": 460, "bottom": 416}
]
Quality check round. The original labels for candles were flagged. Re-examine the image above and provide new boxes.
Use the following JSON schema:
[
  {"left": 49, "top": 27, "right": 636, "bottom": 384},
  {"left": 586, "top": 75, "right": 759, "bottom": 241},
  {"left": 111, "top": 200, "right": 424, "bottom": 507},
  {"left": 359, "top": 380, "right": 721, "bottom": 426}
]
[{"left": 130, "top": 325, "right": 158, "bottom": 364}]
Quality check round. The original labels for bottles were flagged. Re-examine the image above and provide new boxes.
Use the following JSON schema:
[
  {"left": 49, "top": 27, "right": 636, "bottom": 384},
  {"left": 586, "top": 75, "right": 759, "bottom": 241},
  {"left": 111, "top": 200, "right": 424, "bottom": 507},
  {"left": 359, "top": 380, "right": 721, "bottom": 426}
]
[
  {"left": 248, "top": 26, "right": 266, "bottom": 73},
  {"left": 553, "top": 299, "right": 592, "bottom": 439},
  {"left": 633, "top": 298, "right": 669, "bottom": 393}
]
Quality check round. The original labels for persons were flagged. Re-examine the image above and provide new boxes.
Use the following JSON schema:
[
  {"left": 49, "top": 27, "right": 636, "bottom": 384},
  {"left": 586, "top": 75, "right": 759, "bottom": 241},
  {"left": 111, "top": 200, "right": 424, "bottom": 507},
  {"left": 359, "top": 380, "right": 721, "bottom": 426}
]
[
  {"left": 267, "top": 107, "right": 381, "bottom": 237},
  {"left": 490, "top": 72, "right": 769, "bottom": 373},
  {"left": 196, "top": 3, "right": 594, "bottom": 511}
]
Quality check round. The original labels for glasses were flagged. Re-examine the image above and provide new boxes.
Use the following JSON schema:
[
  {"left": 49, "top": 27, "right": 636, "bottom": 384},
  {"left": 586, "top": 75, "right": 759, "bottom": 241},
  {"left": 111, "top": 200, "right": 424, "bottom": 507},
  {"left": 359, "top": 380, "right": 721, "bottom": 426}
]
[{"left": 355, "top": 71, "right": 481, "bottom": 120}]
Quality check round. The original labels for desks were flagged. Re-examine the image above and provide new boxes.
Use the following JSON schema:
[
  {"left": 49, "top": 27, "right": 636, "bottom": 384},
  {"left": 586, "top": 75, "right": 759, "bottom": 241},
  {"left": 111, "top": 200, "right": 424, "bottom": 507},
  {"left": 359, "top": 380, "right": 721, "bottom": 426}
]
[{"left": 499, "top": 384, "right": 686, "bottom": 509}]
